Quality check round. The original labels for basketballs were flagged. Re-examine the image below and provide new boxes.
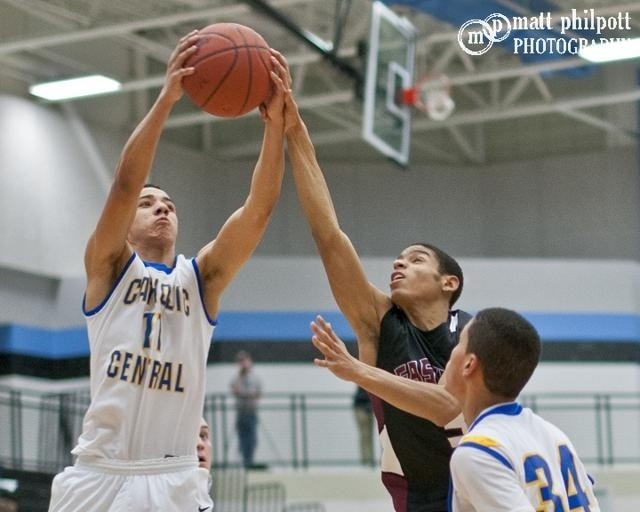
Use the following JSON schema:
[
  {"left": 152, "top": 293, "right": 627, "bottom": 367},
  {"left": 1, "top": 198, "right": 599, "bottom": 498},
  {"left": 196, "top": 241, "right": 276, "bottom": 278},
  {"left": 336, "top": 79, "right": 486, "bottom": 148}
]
[{"left": 181, "top": 22, "right": 276, "bottom": 117}]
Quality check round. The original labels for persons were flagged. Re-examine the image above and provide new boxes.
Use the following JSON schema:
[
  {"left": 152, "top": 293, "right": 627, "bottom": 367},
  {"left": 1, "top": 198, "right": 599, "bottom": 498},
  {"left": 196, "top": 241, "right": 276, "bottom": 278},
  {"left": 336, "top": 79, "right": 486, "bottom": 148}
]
[
  {"left": 46, "top": 28, "right": 294, "bottom": 512},
  {"left": 352, "top": 380, "right": 379, "bottom": 469},
  {"left": 255, "top": 75, "right": 480, "bottom": 512},
  {"left": 230, "top": 349, "right": 267, "bottom": 470},
  {"left": 196, "top": 416, "right": 216, "bottom": 484},
  {"left": 439, "top": 304, "right": 604, "bottom": 512}
]
